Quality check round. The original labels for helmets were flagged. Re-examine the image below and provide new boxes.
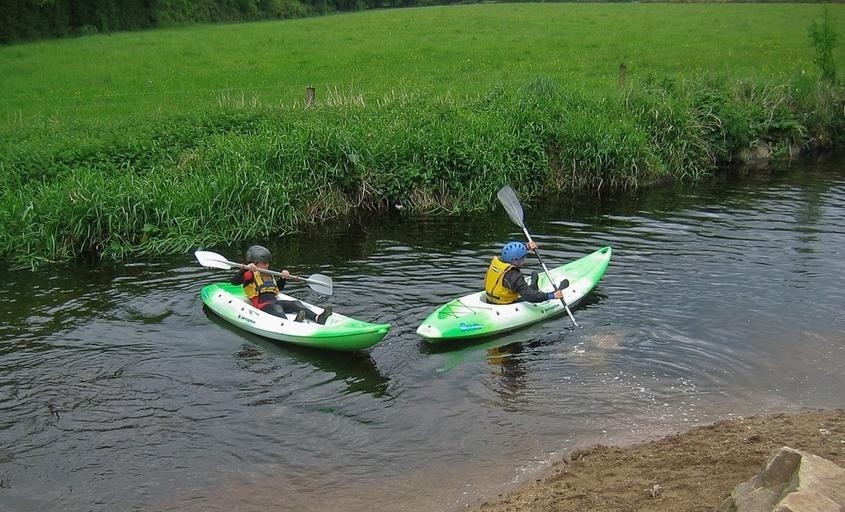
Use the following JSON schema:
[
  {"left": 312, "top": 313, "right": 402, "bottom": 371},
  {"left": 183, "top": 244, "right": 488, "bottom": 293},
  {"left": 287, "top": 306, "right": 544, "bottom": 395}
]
[
  {"left": 246, "top": 245, "right": 272, "bottom": 264},
  {"left": 502, "top": 241, "right": 528, "bottom": 262}
]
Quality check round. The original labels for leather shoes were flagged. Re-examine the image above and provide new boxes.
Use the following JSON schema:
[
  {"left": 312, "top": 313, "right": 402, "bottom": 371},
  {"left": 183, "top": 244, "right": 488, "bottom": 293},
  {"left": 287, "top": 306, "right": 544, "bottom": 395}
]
[
  {"left": 317, "top": 307, "right": 332, "bottom": 325},
  {"left": 294, "top": 309, "right": 305, "bottom": 321},
  {"left": 531, "top": 271, "right": 539, "bottom": 290},
  {"left": 559, "top": 279, "right": 569, "bottom": 290}
]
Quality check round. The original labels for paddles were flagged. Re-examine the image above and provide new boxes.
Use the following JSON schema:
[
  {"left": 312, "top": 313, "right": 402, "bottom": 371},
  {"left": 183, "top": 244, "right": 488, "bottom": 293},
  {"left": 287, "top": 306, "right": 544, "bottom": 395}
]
[
  {"left": 194, "top": 251, "right": 333, "bottom": 296},
  {"left": 498, "top": 185, "right": 578, "bottom": 327}
]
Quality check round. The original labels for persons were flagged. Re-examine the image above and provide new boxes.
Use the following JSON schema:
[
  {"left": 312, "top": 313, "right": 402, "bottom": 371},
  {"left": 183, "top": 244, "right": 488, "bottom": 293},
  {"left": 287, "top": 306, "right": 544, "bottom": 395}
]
[
  {"left": 484, "top": 240, "right": 571, "bottom": 307},
  {"left": 229, "top": 243, "right": 333, "bottom": 328}
]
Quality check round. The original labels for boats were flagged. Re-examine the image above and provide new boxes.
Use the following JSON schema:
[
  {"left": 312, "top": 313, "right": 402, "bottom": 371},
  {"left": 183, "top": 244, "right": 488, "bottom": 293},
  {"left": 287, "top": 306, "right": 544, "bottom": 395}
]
[
  {"left": 201, "top": 277, "right": 392, "bottom": 354},
  {"left": 422, "top": 307, "right": 574, "bottom": 379},
  {"left": 260, "top": 334, "right": 390, "bottom": 398},
  {"left": 415, "top": 246, "right": 614, "bottom": 350}
]
